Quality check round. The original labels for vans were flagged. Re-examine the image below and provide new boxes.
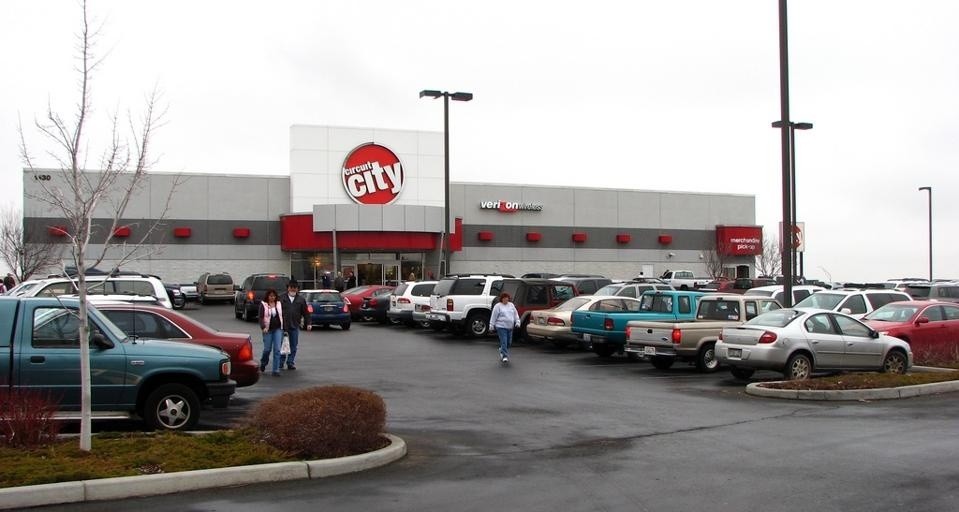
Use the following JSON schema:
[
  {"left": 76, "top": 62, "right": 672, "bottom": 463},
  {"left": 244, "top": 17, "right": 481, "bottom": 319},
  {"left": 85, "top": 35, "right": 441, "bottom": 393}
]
[
  {"left": 701, "top": 281, "right": 734, "bottom": 291},
  {"left": 24, "top": 276, "right": 173, "bottom": 310},
  {"left": 906, "top": 283, "right": 959, "bottom": 300},
  {"left": 732, "top": 279, "right": 778, "bottom": 294},
  {"left": 194, "top": 272, "right": 235, "bottom": 305},
  {"left": 2, "top": 279, "right": 92, "bottom": 299},
  {"left": 735, "top": 285, "right": 826, "bottom": 315}
]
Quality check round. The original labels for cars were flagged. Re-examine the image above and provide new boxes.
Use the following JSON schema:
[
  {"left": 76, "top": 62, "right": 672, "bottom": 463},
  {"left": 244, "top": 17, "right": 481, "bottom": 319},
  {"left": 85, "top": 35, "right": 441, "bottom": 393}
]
[
  {"left": 859, "top": 300, "right": 959, "bottom": 368},
  {"left": 34, "top": 294, "right": 171, "bottom": 337},
  {"left": 299, "top": 289, "right": 351, "bottom": 330},
  {"left": 34, "top": 305, "right": 260, "bottom": 387},
  {"left": 526, "top": 296, "right": 650, "bottom": 353},
  {"left": 412, "top": 299, "right": 435, "bottom": 328},
  {"left": 180, "top": 283, "right": 198, "bottom": 300},
  {"left": 715, "top": 307, "right": 913, "bottom": 381},
  {"left": 358, "top": 289, "right": 401, "bottom": 324},
  {"left": 340, "top": 286, "right": 400, "bottom": 324},
  {"left": 166, "top": 284, "right": 186, "bottom": 309}
]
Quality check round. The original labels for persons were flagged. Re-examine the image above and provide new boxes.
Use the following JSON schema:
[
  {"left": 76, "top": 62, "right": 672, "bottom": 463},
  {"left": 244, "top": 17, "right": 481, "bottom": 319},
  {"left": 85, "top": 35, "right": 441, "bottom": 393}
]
[
  {"left": 347, "top": 271, "right": 356, "bottom": 289},
  {"left": 0, "top": 278, "right": 7, "bottom": 296},
  {"left": 322, "top": 272, "right": 331, "bottom": 288},
  {"left": 277, "top": 280, "right": 313, "bottom": 371},
  {"left": 260, "top": 289, "right": 284, "bottom": 377},
  {"left": 489, "top": 293, "right": 521, "bottom": 365},
  {"left": 3, "top": 273, "right": 15, "bottom": 291},
  {"left": 334, "top": 271, "right": 344, "bottom": 292}
]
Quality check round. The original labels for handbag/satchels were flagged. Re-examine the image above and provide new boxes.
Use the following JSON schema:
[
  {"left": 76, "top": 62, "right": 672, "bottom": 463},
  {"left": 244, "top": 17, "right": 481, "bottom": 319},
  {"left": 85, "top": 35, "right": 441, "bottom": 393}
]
[{"left": 280, "top": 332, "right": 291, "bottom": 355}]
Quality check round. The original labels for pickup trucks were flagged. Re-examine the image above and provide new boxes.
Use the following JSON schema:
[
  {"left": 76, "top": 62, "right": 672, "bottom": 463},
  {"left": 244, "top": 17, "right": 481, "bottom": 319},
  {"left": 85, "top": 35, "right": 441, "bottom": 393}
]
[
  {"left": 659, "top": 271, "right": 714, "bottom": 291},
  {"left": 571, "top": 290, "right": 707, "bottom": 356},
  {"left": 0, "top": 297, "right": 237, "bottom": 430},
  {"left": 624, "top": 294, "right": 785, "bottom": 373}
]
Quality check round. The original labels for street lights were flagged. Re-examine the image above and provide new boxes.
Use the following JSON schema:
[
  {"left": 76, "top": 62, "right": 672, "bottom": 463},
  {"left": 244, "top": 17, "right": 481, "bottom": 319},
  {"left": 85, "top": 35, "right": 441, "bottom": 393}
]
[
  {"left": 919, "top": 186, "right": 931, "bottom": 284},
  {"left": 419, "top": 90, "right": 472, "bottom": 275},
  {"left": 772, "top": 121, "right": 813, "bottom": 279}
]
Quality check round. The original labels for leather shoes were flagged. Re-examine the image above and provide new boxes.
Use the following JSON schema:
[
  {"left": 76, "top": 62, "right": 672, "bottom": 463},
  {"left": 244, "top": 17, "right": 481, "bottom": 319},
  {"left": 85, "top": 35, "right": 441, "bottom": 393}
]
[
  {"left": 272, "top": 372, "right": 280, "bottom": 377},
  {"left": 279, "top": 363, "right": 284, "bottom": 368},
  {"left": 286, "top": 362, "right": 296, "bottom": 369},
  {"left": 260, "top": 361, "right": 265, "bottom": 371}
]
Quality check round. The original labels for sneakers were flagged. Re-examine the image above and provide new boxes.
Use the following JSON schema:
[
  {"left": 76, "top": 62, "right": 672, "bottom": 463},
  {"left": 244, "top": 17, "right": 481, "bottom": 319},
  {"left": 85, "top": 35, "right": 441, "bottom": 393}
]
[
  {"left": 499, "top": 347, "right": 503, "bottom": 360},
  {"left": 502, "top": 357, "right": 508, "bottom": 362}
]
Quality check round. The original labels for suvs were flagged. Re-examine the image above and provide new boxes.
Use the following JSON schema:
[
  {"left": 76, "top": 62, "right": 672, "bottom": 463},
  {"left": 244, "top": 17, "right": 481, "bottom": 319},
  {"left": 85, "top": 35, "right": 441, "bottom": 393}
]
[
  {"left": 430, "top": 274, "right": 517, "bottom": 338},
  {"left": 492, "top": 277, "right": 584, "bottom": 342},
  {"left": 233, "top": 273, "right": 290, "bottom": 321},
  {"left": 794, "top": 284, "right": 917, "bottom": 330},
  {"left": 594, "top": 276, "right": 676, "bottom": 298},
  {"left": 386, "top": 282, "right": 439, "bottom": 328},
  {"left": 561, "top": 275, "right": 611, "bottom": 294}
]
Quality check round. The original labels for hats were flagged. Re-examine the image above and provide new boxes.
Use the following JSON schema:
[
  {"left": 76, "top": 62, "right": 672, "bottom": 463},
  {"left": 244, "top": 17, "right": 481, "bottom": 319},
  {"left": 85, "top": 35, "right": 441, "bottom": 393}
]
[{"left": 286, "top": 280, "right": 300, "bottom": 290}]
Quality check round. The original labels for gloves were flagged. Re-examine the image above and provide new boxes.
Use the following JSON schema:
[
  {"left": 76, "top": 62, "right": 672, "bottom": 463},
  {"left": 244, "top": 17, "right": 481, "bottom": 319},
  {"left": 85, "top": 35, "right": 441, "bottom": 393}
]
[
  {"left": 489, "top": 324, "right": 494, "bottom": 333},
  {"left": 515, "top": 320, "right": 520, "bottom": 328}
]
